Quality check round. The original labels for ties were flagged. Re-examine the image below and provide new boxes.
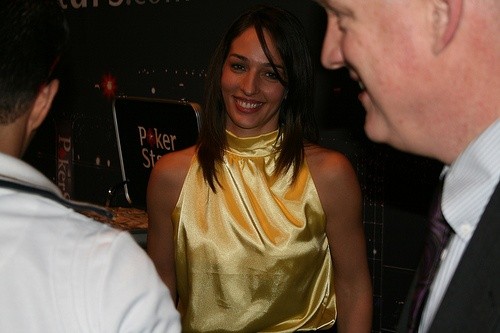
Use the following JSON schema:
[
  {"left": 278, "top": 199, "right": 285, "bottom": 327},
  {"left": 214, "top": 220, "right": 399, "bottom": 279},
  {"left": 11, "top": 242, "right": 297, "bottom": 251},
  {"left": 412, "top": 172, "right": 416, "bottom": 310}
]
[{"left": 395, "top": 172, "right": 455, "bottom": 332}]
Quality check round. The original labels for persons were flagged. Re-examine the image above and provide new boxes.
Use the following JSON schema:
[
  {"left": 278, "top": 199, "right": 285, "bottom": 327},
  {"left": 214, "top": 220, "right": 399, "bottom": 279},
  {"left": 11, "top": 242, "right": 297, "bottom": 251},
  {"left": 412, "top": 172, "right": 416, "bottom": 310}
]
[
  {"left": 315, "top": 0, "right": 500, "bottom": 333},
  {"left": 145, "top": 4, "right": 374, "bottom": 333},
  {"left": 0, "top": 1, "right": 183, "bottom": 333}
]
